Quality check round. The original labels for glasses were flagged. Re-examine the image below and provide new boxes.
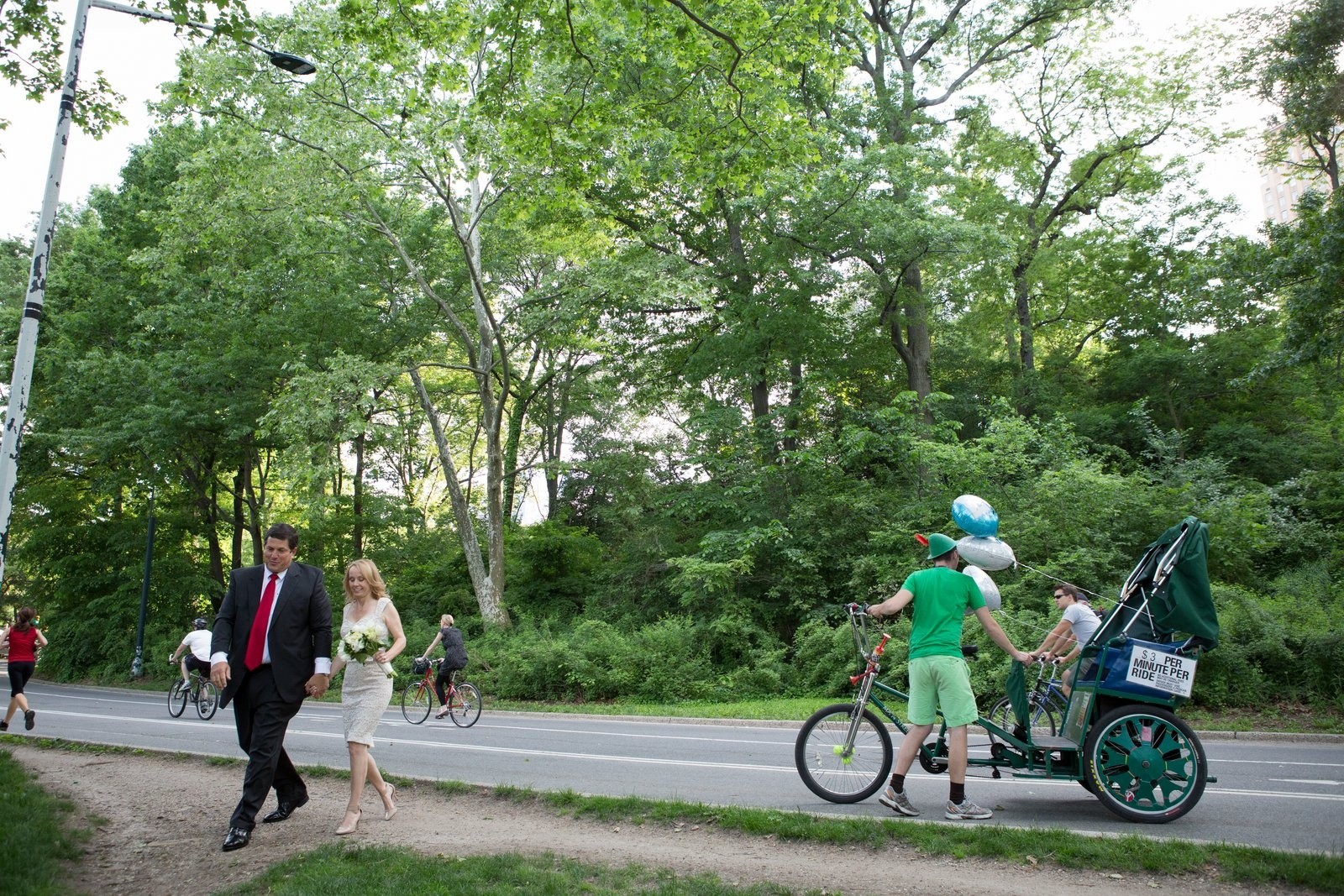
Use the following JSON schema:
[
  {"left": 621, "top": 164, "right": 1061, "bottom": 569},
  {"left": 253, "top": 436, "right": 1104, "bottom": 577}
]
[{"left": 1054, "top": 594, "right": 1068, "bottom": 599}]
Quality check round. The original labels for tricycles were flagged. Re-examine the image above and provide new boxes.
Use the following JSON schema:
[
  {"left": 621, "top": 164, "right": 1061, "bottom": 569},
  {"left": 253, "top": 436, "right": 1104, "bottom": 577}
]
[{"left": 795, "top": 602, "right": 1217, "bottom": 824}]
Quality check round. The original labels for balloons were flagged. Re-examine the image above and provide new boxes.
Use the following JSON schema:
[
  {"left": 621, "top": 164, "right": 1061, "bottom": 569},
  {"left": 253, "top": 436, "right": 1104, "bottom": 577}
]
[
  {"left": 955, "top": 536, "right": 1017, "bottom": 570},
  {"left": 961, "top": 565, "right": 1001, "bottom": 615},
  {"left": 952, "top": 495, "right": 998, "bottom": 538}
]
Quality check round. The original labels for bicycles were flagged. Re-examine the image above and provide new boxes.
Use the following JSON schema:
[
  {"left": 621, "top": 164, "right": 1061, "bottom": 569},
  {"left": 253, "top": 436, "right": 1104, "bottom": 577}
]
[
  {"left": 1003, "top": 655, "right": 1068, "bottom": 755},
  {"left": 167, "top": 653, "right": 219, "bottom": 721},
  {"left": 401, "top": 657, "right": 482, "bottom": 728},
  {"left": 987, "top": 654, "right": 1069, "bottom": 762}
]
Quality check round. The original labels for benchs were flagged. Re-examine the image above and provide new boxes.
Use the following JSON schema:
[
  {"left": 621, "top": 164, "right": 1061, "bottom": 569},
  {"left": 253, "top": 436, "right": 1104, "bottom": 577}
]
[{"left": 1075, "top": 631, "right": 1194, "bottom": 698}]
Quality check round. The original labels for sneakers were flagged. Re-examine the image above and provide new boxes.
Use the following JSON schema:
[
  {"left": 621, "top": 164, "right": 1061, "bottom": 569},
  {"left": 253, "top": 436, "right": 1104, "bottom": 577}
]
[
  {"left": 945, "top": 792, "right": 993, "bottom": 820},
  {"left": 178, "top": 682, "right": 192, "bottom": 691},
  {"left": 201, "top": 702, "right": 208, "bottom": 713},
  {"left": 879, "top": 785, "right": 920, "bottom": 816}
]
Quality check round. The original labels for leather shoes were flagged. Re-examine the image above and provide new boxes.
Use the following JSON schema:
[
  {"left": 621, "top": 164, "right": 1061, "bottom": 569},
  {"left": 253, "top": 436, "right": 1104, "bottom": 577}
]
[
  {"left": 222, "top": 826, "right": 251, "bottom": 850},
  {"left": 264, "top": 791, "right": 309, "bottom": 822}
]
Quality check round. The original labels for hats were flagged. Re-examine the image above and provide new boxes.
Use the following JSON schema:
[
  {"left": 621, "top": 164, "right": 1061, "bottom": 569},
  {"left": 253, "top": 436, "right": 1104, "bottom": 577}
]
[
  {"left": 1077, "top": 593, "right": 1088, "bottom": 601},
  {"left": 927, "top": 533, "right": 957, "bottom": 560}
]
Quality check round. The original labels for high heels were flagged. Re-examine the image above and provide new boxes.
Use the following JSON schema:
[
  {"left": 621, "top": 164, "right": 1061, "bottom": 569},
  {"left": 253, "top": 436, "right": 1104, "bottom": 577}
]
[
  {"left": 336, "top": 804, "right": 363, "bottom": 834},
  {"left": 379, "top": 782, "right": 398, "bottom": 820}
]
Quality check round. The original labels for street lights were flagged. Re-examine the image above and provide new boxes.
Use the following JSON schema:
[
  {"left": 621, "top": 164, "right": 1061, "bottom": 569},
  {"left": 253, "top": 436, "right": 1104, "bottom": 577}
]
[
  {"left": 97, "top": 435, "right": 155, "bottom": 675},
  {"left": 1, "top": 0, "right": 316, "bottom": 576}
]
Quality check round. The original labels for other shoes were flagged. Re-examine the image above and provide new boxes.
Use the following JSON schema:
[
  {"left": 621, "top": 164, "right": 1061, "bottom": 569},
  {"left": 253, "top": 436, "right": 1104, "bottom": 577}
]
[
  {"left": 446, "top": 693, "right": 455, "bottom": 698},
  {"left": 435, "top": 711, "right": 449, "bottom": 719},
  {"left": 0, "top": 719, "right": 8, "bottom": 731},
  {"left": 24, "top": 710, "right": 35, "bottom": 731}
]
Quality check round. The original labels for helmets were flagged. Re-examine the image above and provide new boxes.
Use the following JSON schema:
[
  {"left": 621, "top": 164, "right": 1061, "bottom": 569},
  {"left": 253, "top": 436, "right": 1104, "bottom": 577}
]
[{"left": 192, "top": 618, "right": 207, "bottom": 628}]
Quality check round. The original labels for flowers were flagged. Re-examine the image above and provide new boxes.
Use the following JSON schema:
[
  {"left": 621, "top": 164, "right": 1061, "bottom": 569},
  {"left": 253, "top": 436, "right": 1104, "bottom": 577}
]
[{"left": 343, "top": 628, "right": 398, "bottom": 677}]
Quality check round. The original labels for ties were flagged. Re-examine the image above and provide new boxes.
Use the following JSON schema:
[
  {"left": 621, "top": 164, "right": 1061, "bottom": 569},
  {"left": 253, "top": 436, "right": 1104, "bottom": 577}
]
[{"left": 244, "top": 575, "right": 279, "bottom": 671}]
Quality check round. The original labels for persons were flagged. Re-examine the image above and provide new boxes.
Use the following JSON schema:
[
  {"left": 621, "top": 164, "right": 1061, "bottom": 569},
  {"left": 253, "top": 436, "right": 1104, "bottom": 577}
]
[
  {"left": 1028, "top": 584, "right": 1104, "bottom": 699},
  {"left": 170, "top": 618, "right": 212, "bottom": 713},
  {"left": 305, "top": 559, "right": 406, "bottom": 834},
  {"left": 417, "top": 614, "right": 469, "bottom": 718},
  {"left": 0, "top": 608, "right": 48, "bottom": 731},
  {"left": 209, "top": 524, "right": 332, "bottom": 851},
  {"left": 866, "top": 533, "right": 1033, "bottom": 819}
]
